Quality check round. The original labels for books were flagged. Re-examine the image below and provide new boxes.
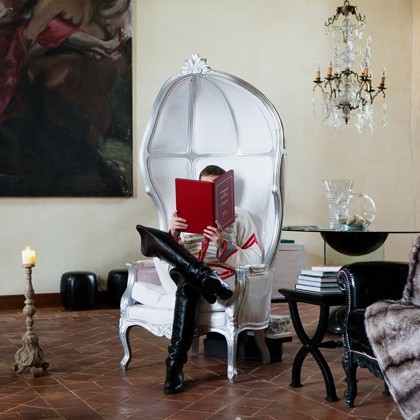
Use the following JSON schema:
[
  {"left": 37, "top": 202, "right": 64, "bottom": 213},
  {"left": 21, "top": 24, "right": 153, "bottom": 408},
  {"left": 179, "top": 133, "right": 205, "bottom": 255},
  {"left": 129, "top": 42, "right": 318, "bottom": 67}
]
[
  {"left": 175, "top": 169, "right": 235, "bottom": 235},
  {"left": 295, "top": 265, "right": 343, "bottom": 293}
]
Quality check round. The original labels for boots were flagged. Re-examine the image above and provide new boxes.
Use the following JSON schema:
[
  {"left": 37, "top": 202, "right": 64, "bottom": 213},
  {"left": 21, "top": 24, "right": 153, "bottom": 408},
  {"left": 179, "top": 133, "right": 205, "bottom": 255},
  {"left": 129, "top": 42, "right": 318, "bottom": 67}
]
[
  {"left": 164, "top": 269, "right": 200, "bottom": 393},
  {"left": 136, "top": 224, "right": 234, "bottom": 300}
]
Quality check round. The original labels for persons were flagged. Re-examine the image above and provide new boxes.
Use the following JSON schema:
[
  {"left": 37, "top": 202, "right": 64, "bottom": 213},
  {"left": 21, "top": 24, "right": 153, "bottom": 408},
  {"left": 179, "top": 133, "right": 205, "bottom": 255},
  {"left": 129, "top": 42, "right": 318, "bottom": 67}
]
[{"left": 135, "top": 165, "right": 263, "bottom": 395}]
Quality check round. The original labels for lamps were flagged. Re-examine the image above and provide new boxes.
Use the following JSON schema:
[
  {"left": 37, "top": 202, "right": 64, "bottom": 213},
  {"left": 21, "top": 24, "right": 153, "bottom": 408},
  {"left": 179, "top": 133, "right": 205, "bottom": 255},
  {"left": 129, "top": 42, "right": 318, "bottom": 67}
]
[{"left": 312, "top": 0, "right": 390, "bottom": 135}]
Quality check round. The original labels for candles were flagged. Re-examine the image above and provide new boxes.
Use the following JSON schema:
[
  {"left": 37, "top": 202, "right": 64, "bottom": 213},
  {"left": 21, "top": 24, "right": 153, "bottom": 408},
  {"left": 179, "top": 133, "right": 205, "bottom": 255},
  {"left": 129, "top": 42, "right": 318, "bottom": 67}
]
[{"left": 21, "top": 246, "right": 35, "bottom": 265}]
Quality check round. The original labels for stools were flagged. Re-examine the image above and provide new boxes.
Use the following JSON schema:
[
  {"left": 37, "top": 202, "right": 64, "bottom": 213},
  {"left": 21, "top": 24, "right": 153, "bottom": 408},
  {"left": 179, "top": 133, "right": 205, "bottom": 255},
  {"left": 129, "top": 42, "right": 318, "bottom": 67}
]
[
  {"left": 106, "top": 269, "right": 127, "bottom": 309},
  {"left": 60, "top": 272, "right": 96, "bottom": 310}
]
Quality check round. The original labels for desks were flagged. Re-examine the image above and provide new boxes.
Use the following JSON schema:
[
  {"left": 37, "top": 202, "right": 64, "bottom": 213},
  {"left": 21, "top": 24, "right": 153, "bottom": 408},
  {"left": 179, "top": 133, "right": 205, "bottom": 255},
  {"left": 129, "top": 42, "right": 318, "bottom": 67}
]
[
  {"left": 281, "top": 225, "right": 420, "bottom": 265},
  {"left": 273, "top": 244, "right": 306, "bottom": 299},
  {"left": 277, "top": 289, "right": 348, "bottom": 402}
]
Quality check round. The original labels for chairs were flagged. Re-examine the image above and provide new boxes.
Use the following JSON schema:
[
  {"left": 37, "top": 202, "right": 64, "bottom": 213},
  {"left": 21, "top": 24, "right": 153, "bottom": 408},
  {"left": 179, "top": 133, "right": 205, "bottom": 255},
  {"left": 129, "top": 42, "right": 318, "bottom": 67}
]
[
  {"left": 336, "top": 235, "right": 420, "bottom": 420},
  {"left": 118, "top": 52, "right": 287, "bottom": 384}
]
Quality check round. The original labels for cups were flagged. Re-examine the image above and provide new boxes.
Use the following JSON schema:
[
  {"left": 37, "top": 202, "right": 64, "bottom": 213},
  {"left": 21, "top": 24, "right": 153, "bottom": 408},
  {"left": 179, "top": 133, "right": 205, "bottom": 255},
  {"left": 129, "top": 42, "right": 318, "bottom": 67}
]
[{"left": 323, "top": 179, "right": 353, "bottom": 231}]
